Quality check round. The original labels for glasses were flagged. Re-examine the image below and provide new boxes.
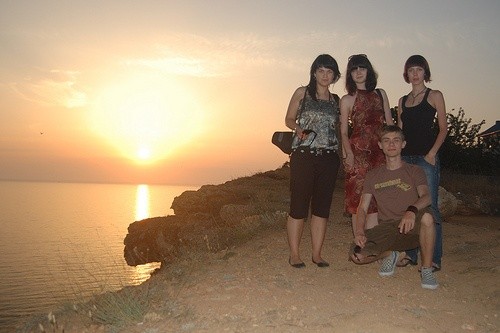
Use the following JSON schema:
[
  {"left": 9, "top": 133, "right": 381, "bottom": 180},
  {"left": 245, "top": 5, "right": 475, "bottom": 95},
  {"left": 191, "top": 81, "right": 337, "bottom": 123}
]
[
  {"left": 353, "top": 240, "right": 376, "bottom": 262},
  {"left": 298, "top": 129, "right": 317, "bottom": 148},
  {"left": 348, "top": 54, "right": 367, "bottom": 62}
]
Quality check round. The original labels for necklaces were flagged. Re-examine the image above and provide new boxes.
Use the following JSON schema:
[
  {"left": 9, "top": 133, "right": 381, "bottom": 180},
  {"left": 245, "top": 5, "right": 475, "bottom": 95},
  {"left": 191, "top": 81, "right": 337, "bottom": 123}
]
[{"left": 410, "top": 85, "right": 427, "bottom": 103}]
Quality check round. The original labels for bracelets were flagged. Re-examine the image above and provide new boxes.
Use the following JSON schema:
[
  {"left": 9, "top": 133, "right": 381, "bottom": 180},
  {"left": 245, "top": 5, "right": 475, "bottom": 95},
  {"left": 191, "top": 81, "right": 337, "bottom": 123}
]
[
  {"left": 342, "top": 157, "right": 346, "bottom": 159},
  {"left": 406, "top": 205, "right": 419, "bottom": 216},
  {"left": 295, "top": 125, "right": 300, "bottom": 132}
]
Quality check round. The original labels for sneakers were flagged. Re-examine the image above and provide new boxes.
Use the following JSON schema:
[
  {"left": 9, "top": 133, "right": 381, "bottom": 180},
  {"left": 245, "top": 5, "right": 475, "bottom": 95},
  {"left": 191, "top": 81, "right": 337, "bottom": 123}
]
[
  {"left": 378, "top": 250, "right": 398, "bottom": 276},
  {"left": 418, "top": 266, "right": 439, "bottom": 290}
]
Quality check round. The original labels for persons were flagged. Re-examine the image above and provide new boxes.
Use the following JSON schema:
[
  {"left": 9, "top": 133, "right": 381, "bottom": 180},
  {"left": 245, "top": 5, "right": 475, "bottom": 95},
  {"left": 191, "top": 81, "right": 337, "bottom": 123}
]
[
  {"left": 285, "top": 53, "right": 348, "bottom": 269},
  {"left": 348, "top": 124, "right": 440, "bottom": 290},
  {"left": 339, "top": 53, "right": 395, "bottom": 240},
  {"left": 397, "top": 54, "right": 449, "bottom": 273}
]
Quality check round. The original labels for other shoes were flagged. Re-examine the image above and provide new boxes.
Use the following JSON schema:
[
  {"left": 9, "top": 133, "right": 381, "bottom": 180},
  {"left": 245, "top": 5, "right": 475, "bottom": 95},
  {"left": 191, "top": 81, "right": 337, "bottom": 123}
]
[
  {"left": 312, "top": 256, "right": 329, "bottom": 267},
  {"left": 289, "top": 256, "right": 306, "bottom": 268}
]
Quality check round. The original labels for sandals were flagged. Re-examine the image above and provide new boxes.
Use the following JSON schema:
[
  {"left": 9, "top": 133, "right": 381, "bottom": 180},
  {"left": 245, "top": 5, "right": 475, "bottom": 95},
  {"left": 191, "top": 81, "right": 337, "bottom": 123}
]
[
  {"left": 395, "top": 256, "right": 417, "bottom": 267},
  {"left": 418, "top": 266, "right": 441, "bottom": 273}
]
